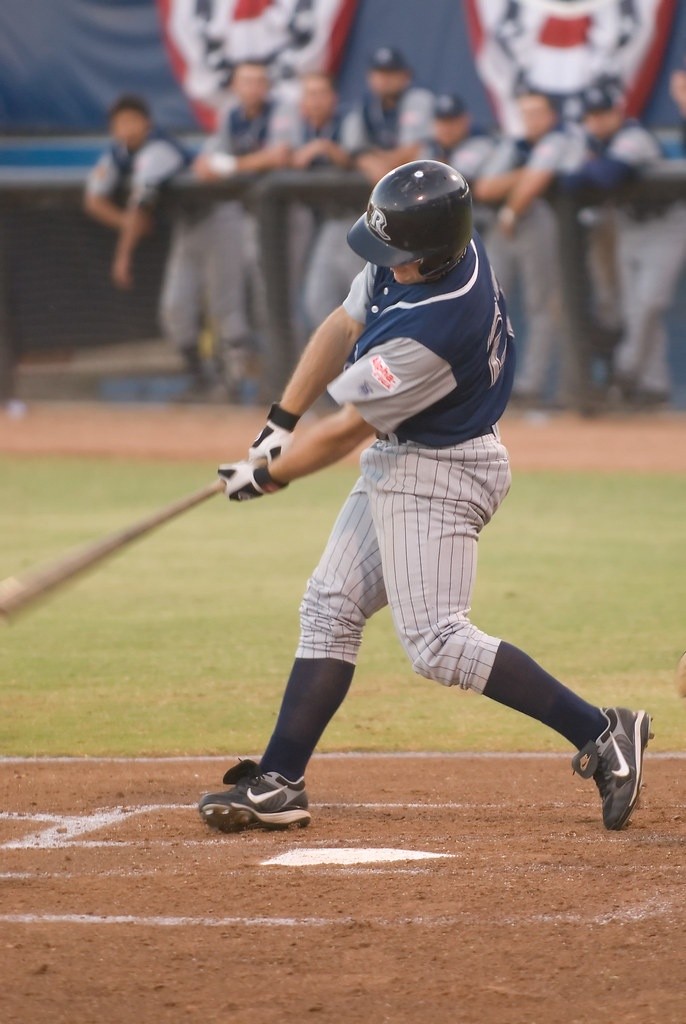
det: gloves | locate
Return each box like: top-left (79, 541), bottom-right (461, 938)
top-left (218, 462), bottom-right (289, 503)
top-left (246, 402), bottom-right (299, 467)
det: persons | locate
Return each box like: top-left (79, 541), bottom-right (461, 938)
top-left (190, 160), bottom-right (650, 832)
top-left (85, 47), bottom-right (686, 426)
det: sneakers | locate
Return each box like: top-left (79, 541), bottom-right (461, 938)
top-left (570, 706), bottom-right (655, 831)
top-left (198, 760), bottom-right (311, 835)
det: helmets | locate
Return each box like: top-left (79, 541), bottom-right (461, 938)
top-left (345, 160), bottom-right (471, 278)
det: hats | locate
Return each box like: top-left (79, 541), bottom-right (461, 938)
top-left (435, 92), bottom-right (464, 120)
top-left (369, 46), bottom-right (404, 72)
top-left (576, 89), bottom-right (610, 125)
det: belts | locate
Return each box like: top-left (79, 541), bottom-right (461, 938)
top-left (375, 426), bottom-right (493, 443)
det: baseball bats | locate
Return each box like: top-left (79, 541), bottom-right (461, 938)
top-left (0, 456), bottom-right (266, 618)
